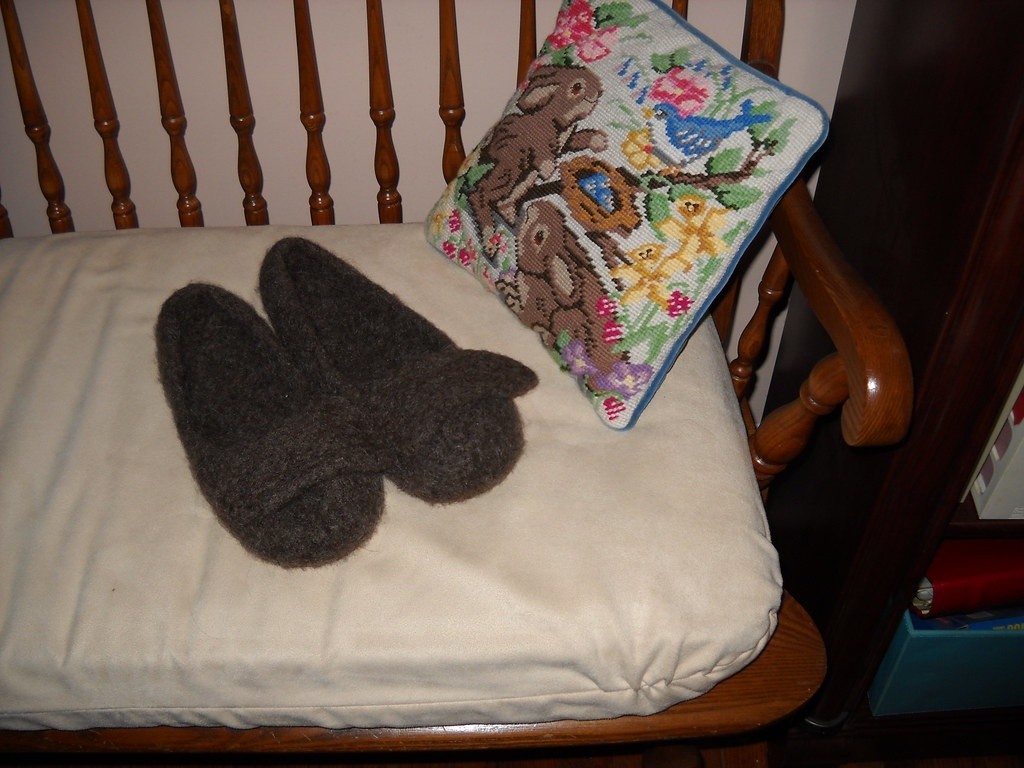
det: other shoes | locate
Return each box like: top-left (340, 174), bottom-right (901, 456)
top-left (156, 282), bottom-right (387, 570)
top-left (257, 236), bottom-right (537, 507)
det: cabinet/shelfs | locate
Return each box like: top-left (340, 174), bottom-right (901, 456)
top-left (763, 0), bottom-right (1024, 768)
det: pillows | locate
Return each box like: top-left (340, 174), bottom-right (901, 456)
top-left (427, 0), bottom-right (835, 433)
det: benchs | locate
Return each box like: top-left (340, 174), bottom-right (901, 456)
top-left (0, 0), bottom-right (919, 768)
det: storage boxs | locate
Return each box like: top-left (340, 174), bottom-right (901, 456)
top-left (870, 602), bottom-right (1024, 718)
top-left (972, 388), bottom-right (1024, 520)
